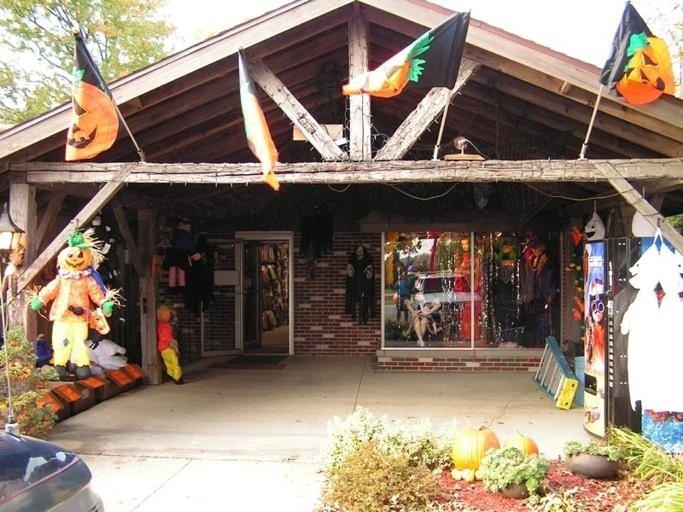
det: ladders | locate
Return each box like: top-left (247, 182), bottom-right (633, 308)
top-left (532, 336), bottom-right (578, 409)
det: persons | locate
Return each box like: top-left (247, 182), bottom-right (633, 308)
top-left (395, 271), bottom-right (409, 325)
top-left (156, 303), bottom-right (185, 385)
top-left (586, 294), bottom-right (605, 376)
top-left (404, 276), bottom-right (441, 346)
top-left (454, 236), bottom-right (482, 337)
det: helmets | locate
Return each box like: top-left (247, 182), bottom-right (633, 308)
top-left (408, 265), bottom-right (416, 270)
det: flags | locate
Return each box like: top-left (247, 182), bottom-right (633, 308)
top-left (599, 1), bottom-right (675, 109)
top-left (62, 31), bottom-right (125, 163)
top-left (341, 4), bottom-right (469, 100)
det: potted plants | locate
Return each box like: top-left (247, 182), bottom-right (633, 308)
top-left (563, 442), bottom-right (621, 478)
top-left (481, 447), bottom-right (549, 506)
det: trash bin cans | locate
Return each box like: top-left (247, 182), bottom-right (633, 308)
top-left (574, 356), bottom-right (584, 407)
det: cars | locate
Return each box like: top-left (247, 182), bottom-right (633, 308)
top-left (399, 269), bottom-right (482, 333)
top-left (0, 429), bottom-right (103, 511)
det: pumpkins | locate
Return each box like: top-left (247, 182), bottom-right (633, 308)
top-left (451, 426), bottom-right (499, 471)
top-left (505, 429), bottom-right (540, 458)
